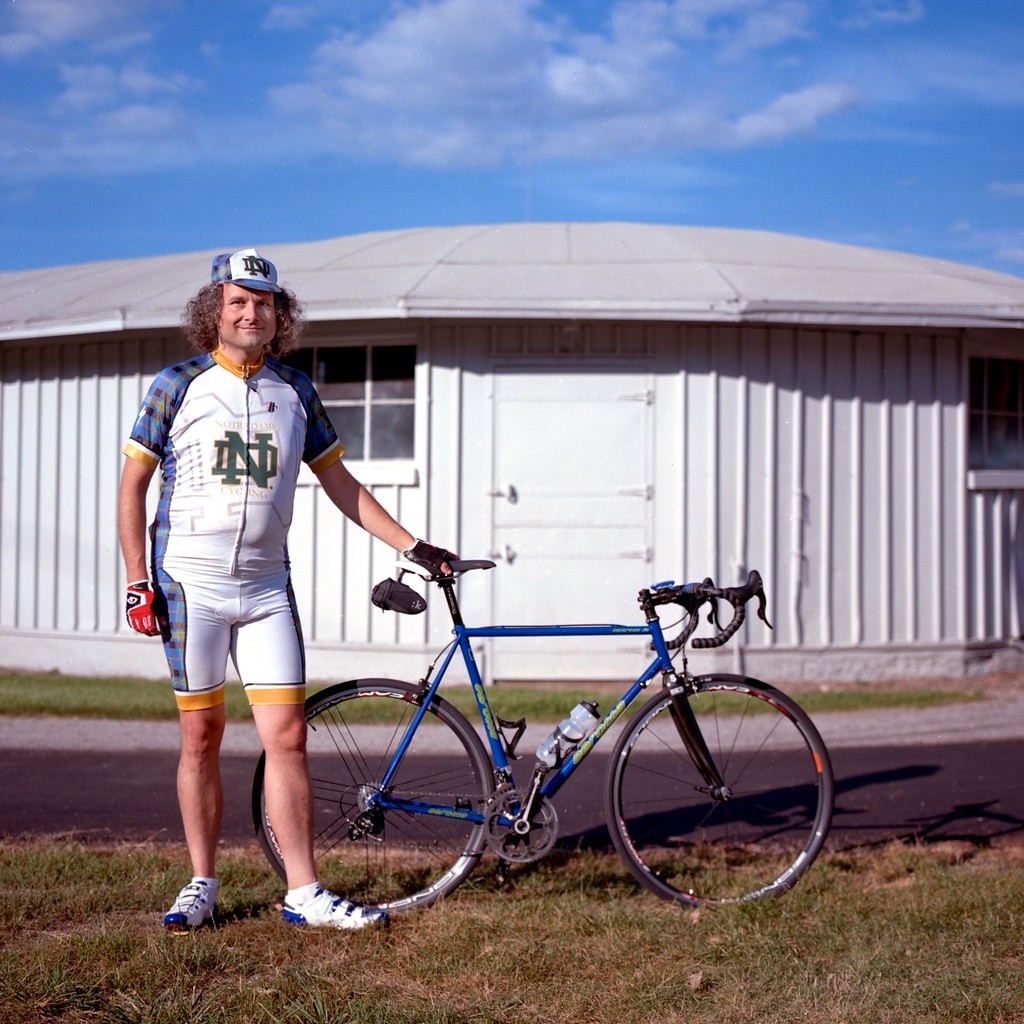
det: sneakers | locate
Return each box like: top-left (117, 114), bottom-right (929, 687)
top-left (283, 887), bottom-right (390, 932)
top-left (164, 882), bottom-right (219, 935)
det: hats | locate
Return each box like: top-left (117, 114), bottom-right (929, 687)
top-left (210, 248), bottom-right (283, 293)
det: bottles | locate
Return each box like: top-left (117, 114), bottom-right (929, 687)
top-left (535, 700), bottom-right (602, 767)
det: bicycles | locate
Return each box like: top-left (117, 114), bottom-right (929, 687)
top-left (252, 558), bottom-right (835, 911)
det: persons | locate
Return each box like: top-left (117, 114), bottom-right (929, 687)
top-left (114, 248), bottom-right (453, 939)
